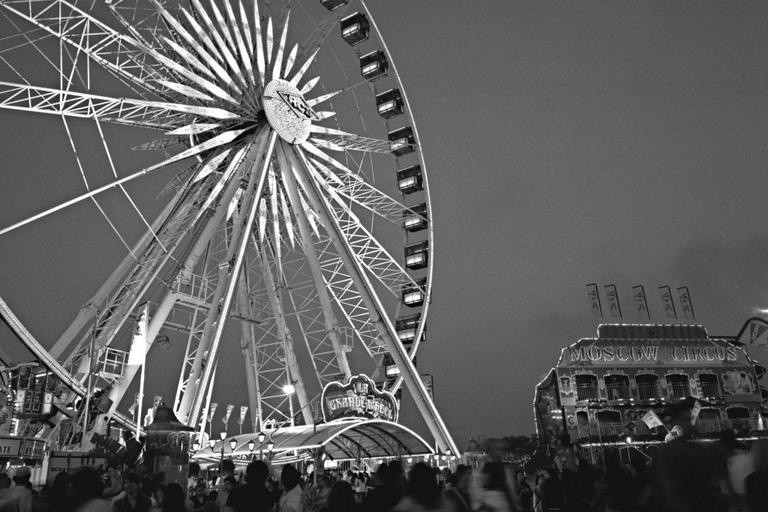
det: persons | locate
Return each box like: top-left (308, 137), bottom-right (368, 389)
top-left (1, 428), bottom-right (767, 511)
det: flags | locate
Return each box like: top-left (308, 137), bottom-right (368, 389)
top-left (151, 394), bottom-right (162, 418)
top-left (237, 406), bottom-right (249, 424)
top-left (207, 404), bottom-right (219, 422)
top-left (222, 403), bottom-right (235, 423)
top-left (128, 392), bottom-right (145, 414)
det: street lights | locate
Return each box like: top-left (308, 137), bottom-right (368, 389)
top-left (188, 440), bottom-right (200, 460)
top-left (281, 385), bottom-right (299, 458)
top-left (209, 402), bottom-right (219, 438)
top-left (209, 432), bottom-right (237, 474)
top-left (253, 407), bottom-right (262, 432)
top-left (246, 433), bottom-right (274, 463)
top-left (238, 406), bottom-right (248, 433)
top-left (223, 404), bottom-right (235, 432)
